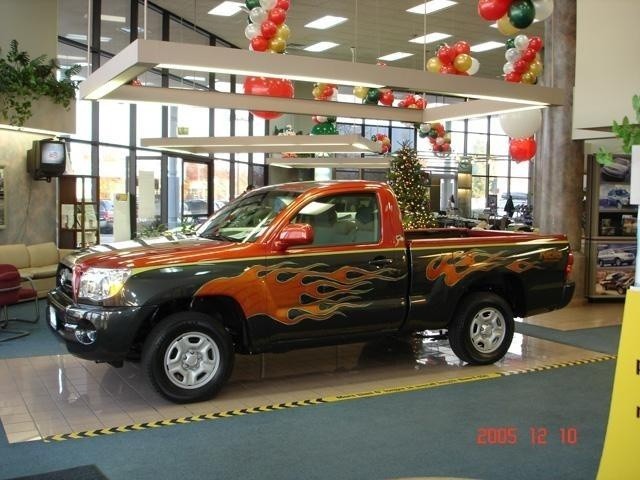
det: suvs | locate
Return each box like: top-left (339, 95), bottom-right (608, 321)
top-left (607, 188), bottom-right (635, 208)
top-left (598, 249), bottom-right (635, 267)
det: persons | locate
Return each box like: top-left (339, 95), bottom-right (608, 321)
top-left (504, 196), bottom-right (515, 218)
top-left (236, 185), bottom-right (255, 198)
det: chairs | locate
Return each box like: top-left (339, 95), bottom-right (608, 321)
top-left (0, 263), bottom-right (42, 329)
top-left (0, 270), bottom-right (31, 342)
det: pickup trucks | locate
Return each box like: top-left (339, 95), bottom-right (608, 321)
top-left (46, 180), bottom-right (575, 405)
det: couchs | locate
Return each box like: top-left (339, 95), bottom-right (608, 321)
top-left (0, 241), bottom-right (83, 304)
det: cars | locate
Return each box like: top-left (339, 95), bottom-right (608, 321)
top-left (600, 157), bottom-right (631, 182)
top-left (98, 197), bottom-right (230, 234)
top-left (599, 272), bottom-right (634, 295)
top-left (497, 191), bottom-right (528, 212)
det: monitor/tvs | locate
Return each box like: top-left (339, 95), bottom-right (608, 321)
top-left (27, 140), bottom-right (66, 177)
